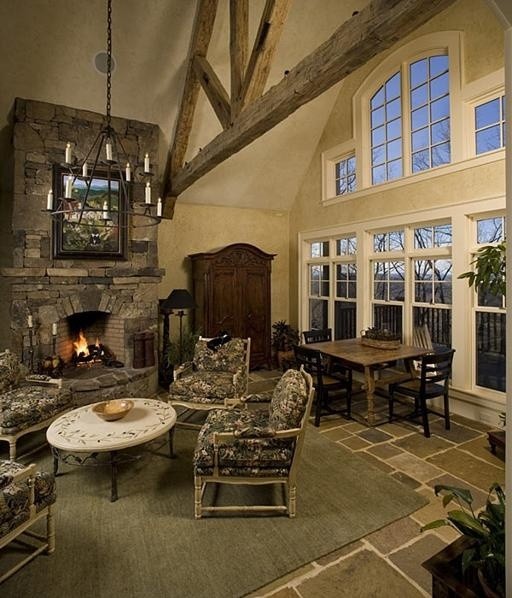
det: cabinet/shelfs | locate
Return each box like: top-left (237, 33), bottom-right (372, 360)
top-left (185, 241), bottom-right (280, 372)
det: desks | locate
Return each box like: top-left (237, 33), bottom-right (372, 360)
top-left (300, 338), bottom-right (435, 429)
top-left (44, 397), bottom-right (178, 502)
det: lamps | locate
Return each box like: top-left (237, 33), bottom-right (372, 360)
top-left (162, 288), bottom-right (199, 367)
top-left (41, 0), bottom-right (166, 230)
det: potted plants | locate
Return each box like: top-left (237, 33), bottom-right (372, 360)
top-left (413, 475), bottom-right (507, 598)
top-left (270, 315), bottom-right (301, 371)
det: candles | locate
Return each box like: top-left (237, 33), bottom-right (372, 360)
top-left (26, 312), bottom-right (33, 326)
top-left (51, 319), bottom-right (57, 336)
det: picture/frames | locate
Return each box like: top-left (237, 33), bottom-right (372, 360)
top-left (48, 163), bottom-right (131, 263)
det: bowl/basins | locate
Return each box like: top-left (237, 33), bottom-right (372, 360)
top-left (92, 397), bottom-right (136, 422)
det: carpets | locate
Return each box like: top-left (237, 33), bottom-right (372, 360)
top-left (1, 426), bottom-right (429, 598)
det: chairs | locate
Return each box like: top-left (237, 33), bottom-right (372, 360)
top-left (384, 348), bottom-right (458, 439)
top-left (166, 332), bottom-right (252, 432)
top-left (293, 342), bottom-right (353, 430)
top-left (301, 328), bottom-right (348, 378)
top-left (0, 454), bottom-right (61, 585)
top-left (0, 348), bottom-right (73, 467)
top-left (183, 363), bottom-right (317, 519)
